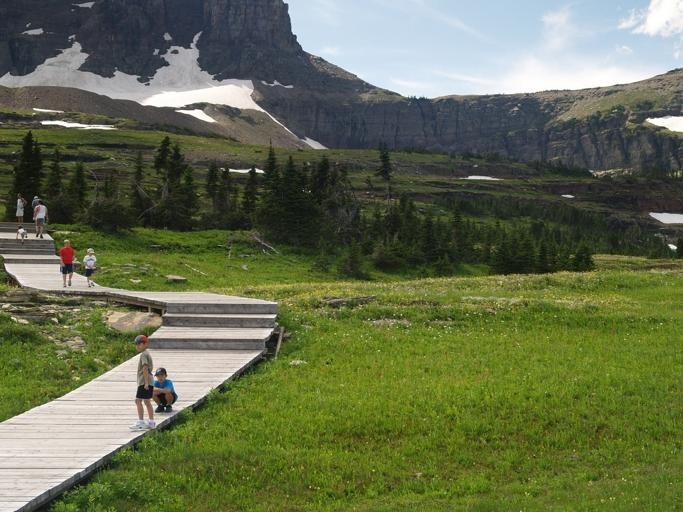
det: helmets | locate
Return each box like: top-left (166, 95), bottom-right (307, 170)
top-left (134, 335), bottom-right (148, 344)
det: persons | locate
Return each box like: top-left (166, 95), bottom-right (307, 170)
top-left (32, 199), bottom-right (48, 239)
top-left (83, 247), bottom-right (97, 287)
top-left (151, 366), bottom-right (177, 414)
top-left (128, 334), bottom-right (157, 432)
top-left (14, 193), bottom-right (28, 223)
top-left (15, 225), bottom-right (28, 246)
top-left (58, 239), bottom-right (76, 287)
top-left (30, 196), bottom-right (41, 210)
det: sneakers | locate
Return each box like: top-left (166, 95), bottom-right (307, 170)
top-left (36, 232), bottom-right (43, 238)
top-left (128, 423), bottom-right (144, 431)
top-left (88, 282), bottom-right (94, 287)
top-left (154, 405), bottom-right (164, 412)
top-left (143, 422), bottom-right (156, 429)
top-left (165, 404), bottom-right (172, 411)
top-left (62, 282), bottom-right (72, 287)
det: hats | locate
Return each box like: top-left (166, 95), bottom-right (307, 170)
top-left (87, 248), bottom-right (95, 254)
top-left (154, 368), bottom-right (166, 376)
